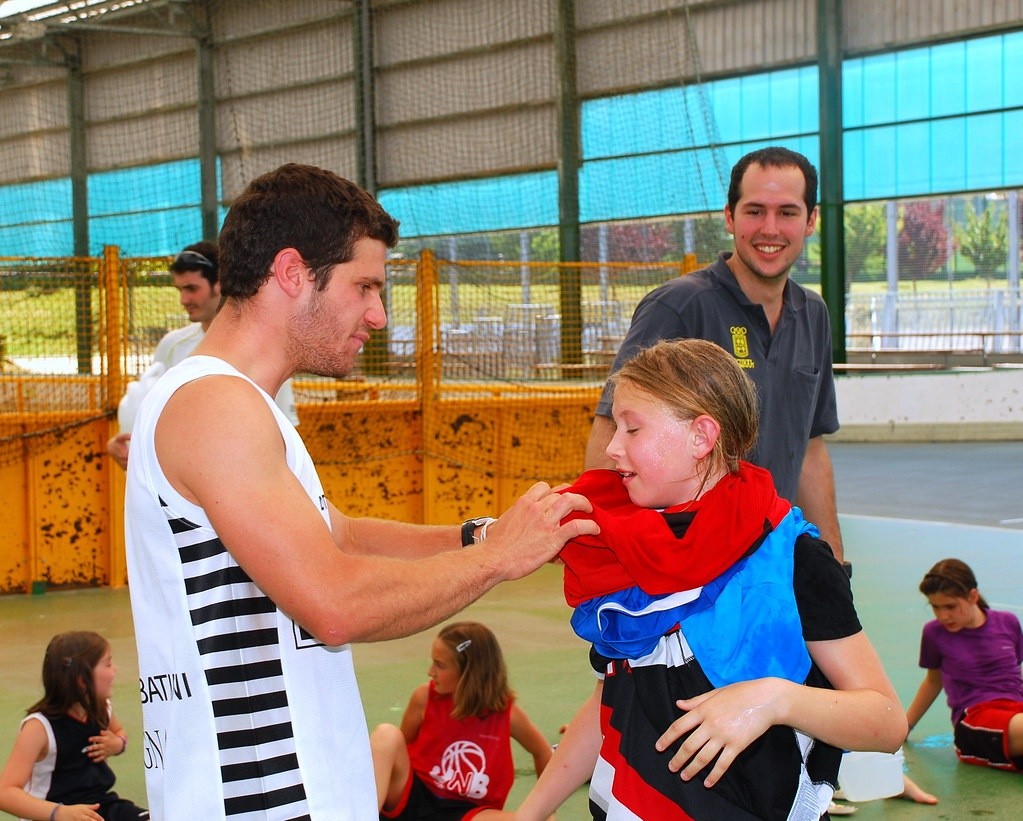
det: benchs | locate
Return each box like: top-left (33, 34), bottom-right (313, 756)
top-left (536, 362), bottom-right (612, 375)
top-left (595, 334), bottom-right (627, 342)
top-left (580, 349), bottom-right (621, 359)
top-left (831, 361), bottom-right (948, 371)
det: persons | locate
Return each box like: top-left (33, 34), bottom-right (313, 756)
top-left (371, 622), bottom-right (560, 821)
top-left (102, 242), bottom-right (305, 472)
top-left (897, 556), bottom-right (1021, 772)
top-left (124, 158), bottom-right (599, 818)
top-left (517, 339), bottom-right (912, 817)
top-left (2, 631), bottom-right (148, 818)
top-left (585, 149), bottom-right (851, 816)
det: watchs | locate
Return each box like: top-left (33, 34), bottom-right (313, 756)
top-left (455, 513), bottom-right (494, 547)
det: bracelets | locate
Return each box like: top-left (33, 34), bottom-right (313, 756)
top-left (479, 518), bottom-right (497, 540)
